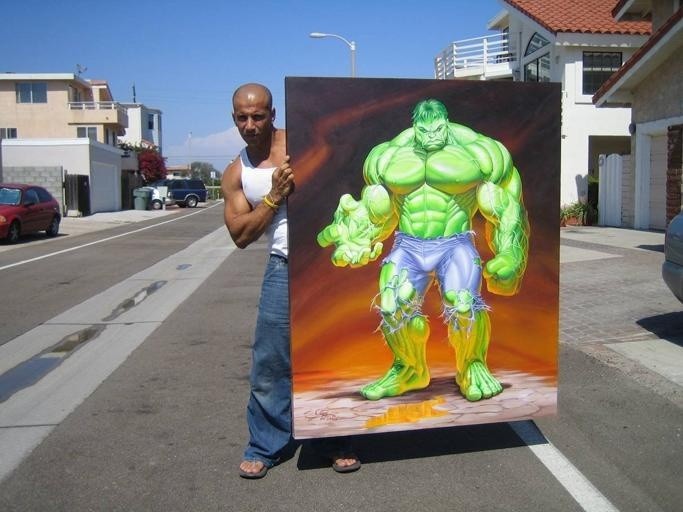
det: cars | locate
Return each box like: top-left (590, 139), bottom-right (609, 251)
top-left (662, 208), bottom-right (683, 304)
top-left (0, 182), bottom-right (61, 242)
top-left (143, 179), bottom-right (208, 209)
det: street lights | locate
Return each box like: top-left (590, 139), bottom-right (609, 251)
top-left (309, 33), bottom-right (356, 77)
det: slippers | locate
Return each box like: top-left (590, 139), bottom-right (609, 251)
top-left (238, 459), bottom-right (268, 479)
top-left (332, 454), bottom-right (360, 472)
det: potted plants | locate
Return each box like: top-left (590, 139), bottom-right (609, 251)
top-left (560, 200), bottom-right (597, 228)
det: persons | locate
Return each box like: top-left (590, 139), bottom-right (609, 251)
top-left (317, 97), bottom-right (531, 402)
top-left (219, 83), bottom-right (361, 478)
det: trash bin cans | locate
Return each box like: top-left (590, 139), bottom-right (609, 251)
top-left (133, 189), bottom-right (152, 210)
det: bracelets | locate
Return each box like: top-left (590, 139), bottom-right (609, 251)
top-left (264, 196), bottom-right (279, 209)
